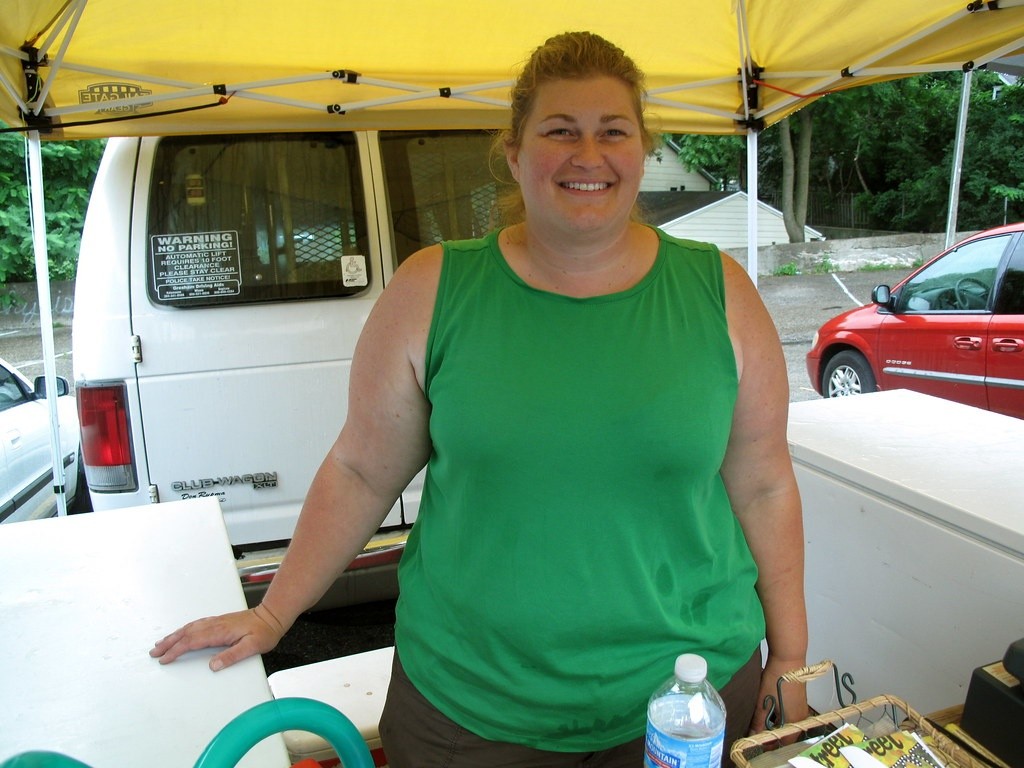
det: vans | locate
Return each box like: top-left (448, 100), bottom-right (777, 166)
top-left (67, 128), bottom-right (531, 616)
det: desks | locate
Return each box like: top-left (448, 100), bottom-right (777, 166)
top-left (0, 493), bottom-right (291, 767)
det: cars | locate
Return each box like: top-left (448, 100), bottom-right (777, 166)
top-left (0, 361), bottom-right (90, 525)
top-left (801, 224), bottom-right (1022, 422)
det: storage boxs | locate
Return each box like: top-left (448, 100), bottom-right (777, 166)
top-left (729, 661), bottom-right (989, 768)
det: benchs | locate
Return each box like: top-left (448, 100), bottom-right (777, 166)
top-left (268, 644), bottom-right (396, 768)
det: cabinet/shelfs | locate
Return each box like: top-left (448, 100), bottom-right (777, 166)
top-left (761, 388), bottom-right (1024, 732)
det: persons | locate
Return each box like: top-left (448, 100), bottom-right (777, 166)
top-left (151, 33), bottom-right (808, 767)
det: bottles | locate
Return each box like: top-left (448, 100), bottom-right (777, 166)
top-left (643, 652), bottom-right (727, 768)
